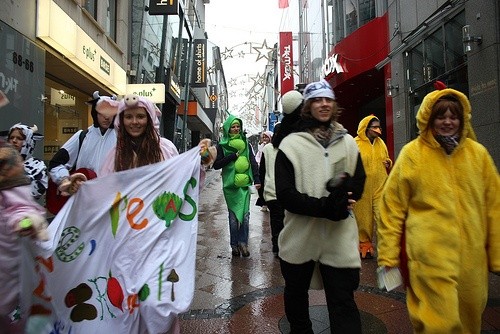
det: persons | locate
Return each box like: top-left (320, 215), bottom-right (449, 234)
top-left (50, 91), bottom-right (118, 188)
top-left (378, 81), bottom-right (500, 334)
top-left (274, 81), bottom-right (367, 334)
top-left (0, 124), bottom-right (46, 334)
top-left (71, 95), bottom-right (217, 334)
top-left (213, 115), bottom-right (261, 258)
top-left (255, 91), bottom-right (304, 260)
top-left (354, 114), bottom-right (392, 259)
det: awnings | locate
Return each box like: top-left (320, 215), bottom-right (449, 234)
top-left (323, 16), bottom-right (387, 90)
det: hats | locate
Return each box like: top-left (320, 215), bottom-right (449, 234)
top-left (303, 82), bottom-right (335, 103)
top-left (282, 90), bottom-right (303, 114)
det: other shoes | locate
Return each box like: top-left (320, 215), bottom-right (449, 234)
top-left (231, 245), bottom-right (240, 259)
top-left (240, 243), bottom-right (251, 258)
top-left (261, 206), bottom-right (267, 212)
